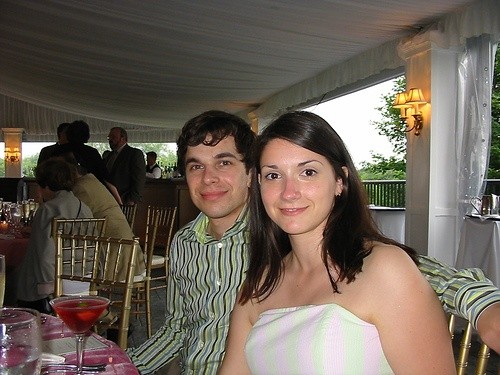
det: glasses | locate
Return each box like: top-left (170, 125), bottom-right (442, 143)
top-left (106, 135), bottom-right (121, 140)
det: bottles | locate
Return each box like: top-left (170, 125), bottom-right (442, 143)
top-left (157, 161), bottom-right (179, 179)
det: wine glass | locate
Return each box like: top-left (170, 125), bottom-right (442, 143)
top-left (49, 295), bottom-right (111, 375)
top-left (0, 198), bottom-right (35, 235)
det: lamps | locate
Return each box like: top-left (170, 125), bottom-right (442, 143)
top-left (3, 146), bottom-right (22, 165)
top-left (390, 88), bottom-right (429, 135)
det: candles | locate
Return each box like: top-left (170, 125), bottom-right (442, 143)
top-left (0, 221), bottom-right (9, 234)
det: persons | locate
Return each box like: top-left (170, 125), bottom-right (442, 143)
top-left (37, 121), bottom-right (102, 180)
top-left (51, 152), bottom-right (143, 334)
top-left (102, 127), bottom-right (145, 204)
top-left (7, 159), bottom-right (98, 301)
top-left (217, 111), bottom-right (457, 375)
top-left (123, 110), bottom-right (500, 375)
top-left (145, 152), bottom-right (162, 179)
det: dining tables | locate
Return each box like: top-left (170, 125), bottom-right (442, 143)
top-left (0, 308), bottom-right (138, 375)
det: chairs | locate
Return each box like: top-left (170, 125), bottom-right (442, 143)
top-left (51, 197), bottom-right (179, 349)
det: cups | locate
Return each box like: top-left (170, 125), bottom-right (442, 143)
top-left (0, 221), bottom-right (9, 234)
top-left (0, 254), bottom-right (6, 308)
top-left (0, 308), bottom-right (41, 375)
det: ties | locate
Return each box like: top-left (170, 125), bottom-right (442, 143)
top-left (108, 152), bottom-right (116, 168)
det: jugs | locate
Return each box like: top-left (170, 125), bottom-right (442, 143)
top-left (470, 194), bottom-right (500, 215)
